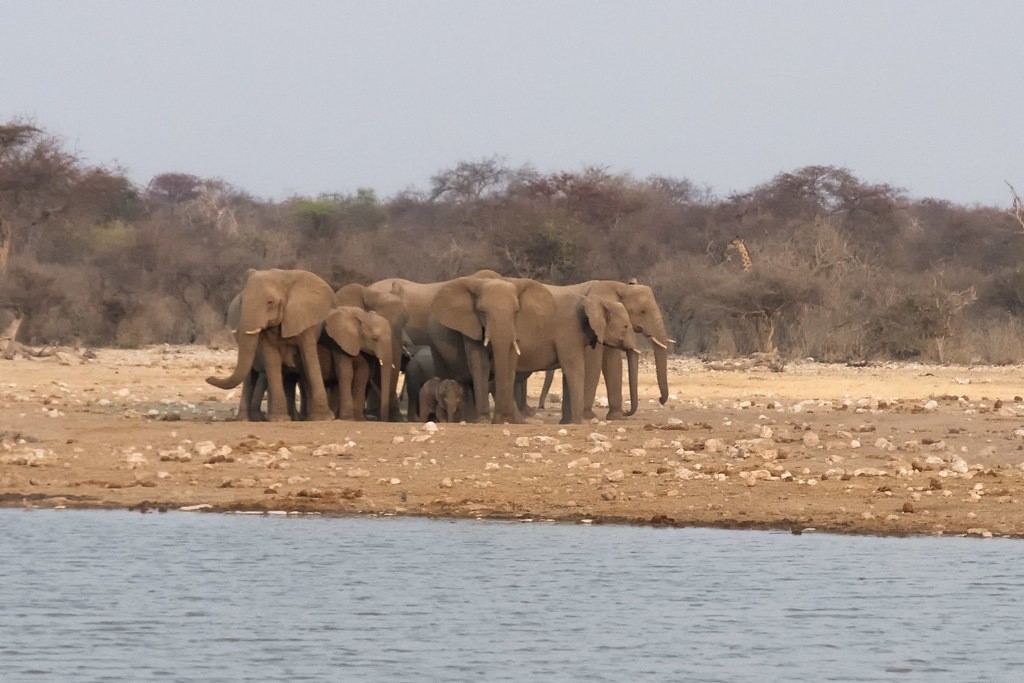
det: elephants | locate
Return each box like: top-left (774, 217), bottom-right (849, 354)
top-left (205, 267), bottom-right (676, 424)
